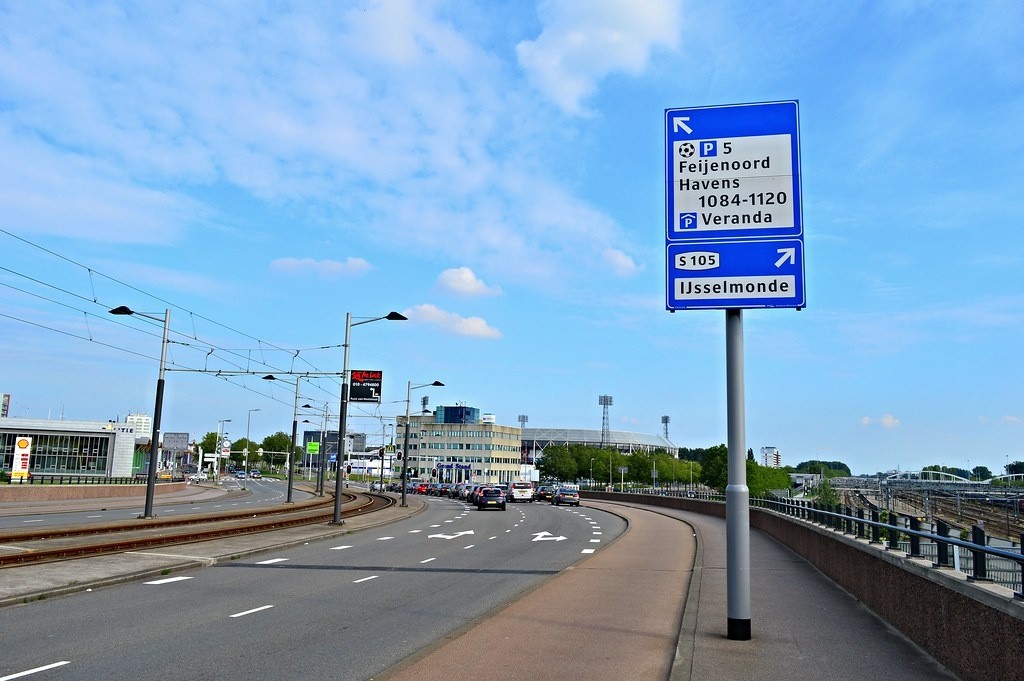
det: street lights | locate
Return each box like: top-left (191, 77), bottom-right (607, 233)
top-left (649, 458), bottom-right (660, 489)
top-left (685, 460), bottom-right (699, 491)
top-left (1006, 454), bottom-right (1010, 486)
top-left (213, 418), bottom-right (232, 479)
top-left (217, 432), bottom-right (229, 459)
top-left (261, 374), bottom-right (300, 507)
top-left (301, 404), bottom-right (329, 497)
top-left (388, 423), bottom-right (394, 473)
top-left (420, 395), bottom-right (429, 416)
top-left (107, 306), bottom-right (171, 519)
top-left (590, 458), bottom-right (595, 492)
top-left (518, 415), bottom-right (529, 428)
top-left (662, 415), bottom-right (670, 439)
top-left (967, 458), bottom-right (971, 487)
top-left (598, 393), bottom-right (613, 450)
top-left (244, 408), bottom-right (261, 490)
top-left (302, 420), bottom-right (323, 493)
top-left (329, 311), bottom-right (408, 525)
top-left (398, 379), bottom-right (446, 507)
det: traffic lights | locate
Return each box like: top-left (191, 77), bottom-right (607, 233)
top-left (432, 468), bottom-right (436, 477)
top-left (347, 466), bottom-right (351, 473)
top-left (379, 449), bottom-right (383, 457)
top-left (397, 452), bottom-right (401, 460)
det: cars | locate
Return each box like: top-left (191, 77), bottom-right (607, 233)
top-left (184, 469), bottom-right (191, 474)
top-left (253, 472), bottom-right (263, 479)
top-left (228, 463), bottom-right (245, 479)
top-left (387, 480), bottom-right (580, 511)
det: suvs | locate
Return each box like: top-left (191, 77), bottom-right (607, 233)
top-left (189, 472), bottom-right (207, 481)
top-left (370, 480), bottom-right (385, 492)
top-left (250, 469), bottom-right (260, 478)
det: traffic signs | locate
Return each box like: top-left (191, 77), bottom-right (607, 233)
top-left (665, 239), bottom-right (807, 310)
top-left (663, 97), bottom-right (804, 242)
top-left (350, 369), bottom-right (383, 403)
top-left (307, 442), bottom-right (319, 454)
